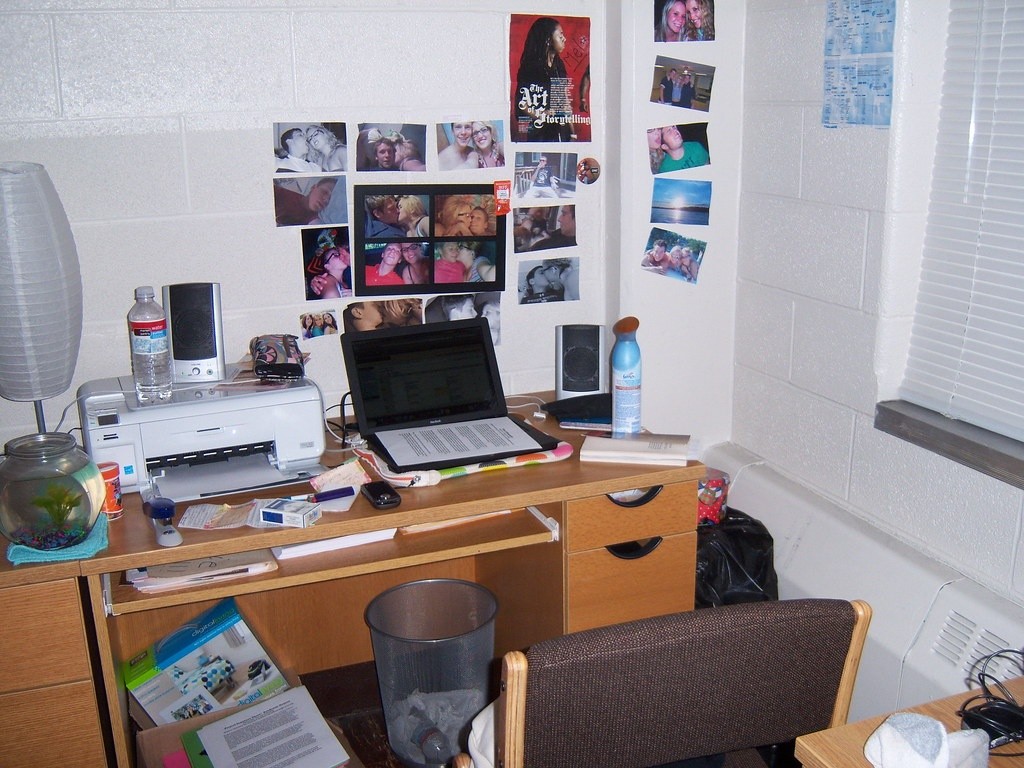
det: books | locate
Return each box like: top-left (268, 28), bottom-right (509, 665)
top-left (543, 392), bottom-right (692, 466)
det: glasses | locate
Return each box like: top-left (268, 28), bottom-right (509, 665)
top-left (307, 129), bottom-right (325, 143)
top-left (401, 244), bottom-right (422, 253)
top-left (539, 160), bottom-right (546, 162)
top-left (647, 127), bottom-right (663, 133)
top-left (471, 125), bottom-right (489, 138)
top-left (457, 213), bottom-right (472, 218)
top-left (671, 254), bottom-right (681, 260)
top-left (682, 254), bottom-right (690, 260)
top-left (322, 252), bottom-right (340, 265)
top-left (459, 244), bottom-right (472, 251)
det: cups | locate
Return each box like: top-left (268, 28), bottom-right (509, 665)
top-left (96, 462), bottom-right (125, 522)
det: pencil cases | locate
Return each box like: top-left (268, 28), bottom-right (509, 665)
top-left (249, 333), bottom-right (305, 382)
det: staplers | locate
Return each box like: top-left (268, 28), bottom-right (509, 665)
top-left (142, 497), bottom-right (184, 548)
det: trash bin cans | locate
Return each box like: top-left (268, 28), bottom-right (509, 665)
top-left (363, 577), bottom-right (499, 768)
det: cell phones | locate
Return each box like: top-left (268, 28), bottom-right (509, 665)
top-left (361, 480), bottom-right (401, 509)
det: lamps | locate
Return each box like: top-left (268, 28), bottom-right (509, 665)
top-left (0, 161), bottom-right (85, 433)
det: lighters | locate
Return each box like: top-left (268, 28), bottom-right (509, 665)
top-left (307, 486), bottom-right (354, 502)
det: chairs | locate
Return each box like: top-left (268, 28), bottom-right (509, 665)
top-left (453, 600), bottom-right (871, 768)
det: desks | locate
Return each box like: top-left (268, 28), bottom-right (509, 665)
top-left (0, 390), bottom-right (705, 768)
top-left (795, 674), bottom-right (1024, 768)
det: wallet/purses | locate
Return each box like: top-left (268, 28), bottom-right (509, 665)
top-left (251, 331), bottom-right (306, 383)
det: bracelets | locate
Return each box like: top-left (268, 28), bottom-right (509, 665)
top-left (581, 99), bottom-right (586, 101)
top-left (570, 135), bottom-right (577, 139)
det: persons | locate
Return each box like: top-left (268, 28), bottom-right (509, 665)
top-left (647, 125), bottom-right (709, 173)
top-left (438, 121), bottom-right (505, 171)
top-left (641, 240), bottom-right (704, 280)
top-left (300, 312), bottom-right (337, 339)
top-left (514, 205), bottom-right (576, 252)
top-left (656, 0), bottom-right (714, 41)
top-left (366, 243), bottom-right (430, 286)
top-left (274, 125), bottom-right (346, 173)
top-left (344, 293), bottom-right (500, 346)
top-left (658, 69), bottom-right (695, 109)
top-left (518, 258), bottom-right (579, 304)
top-left (579, 65), bottom-right (589, 112)
top-left (525, 156), bottom-right (559, 199)
top-left (515, 18), bottom-right (577, 142)
top-left (275, 177), bottom-right (337, 225)
top-left (435, 196), bottom-right (496, 283)
top-left (365, 194), bottom-right (429, 238)
top-left (310, 229), bottom-right (352, 299)
top-left (374, 138), bottom-right (425, 171)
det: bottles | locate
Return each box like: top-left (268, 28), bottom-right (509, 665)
top-left (408, 705), bottom-right (454, 764)
top-left (611, 314), bottom-right (643, 435)
top-left (127, 286), bottom-right (174, 404)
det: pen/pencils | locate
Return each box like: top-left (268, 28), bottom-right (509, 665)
top-left (280, 494), bottom-right (316, 501)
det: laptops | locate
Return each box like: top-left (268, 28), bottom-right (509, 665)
top-left (341, 317), bottom-right (557, 475)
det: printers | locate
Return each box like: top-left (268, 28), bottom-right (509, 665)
top-left (76, 361), bottom-right (333, 504)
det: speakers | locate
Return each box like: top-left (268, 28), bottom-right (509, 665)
top-left (161, 282), bottom-right (227, 384)
top-left (555, 324), bottom-right (606, 401)
